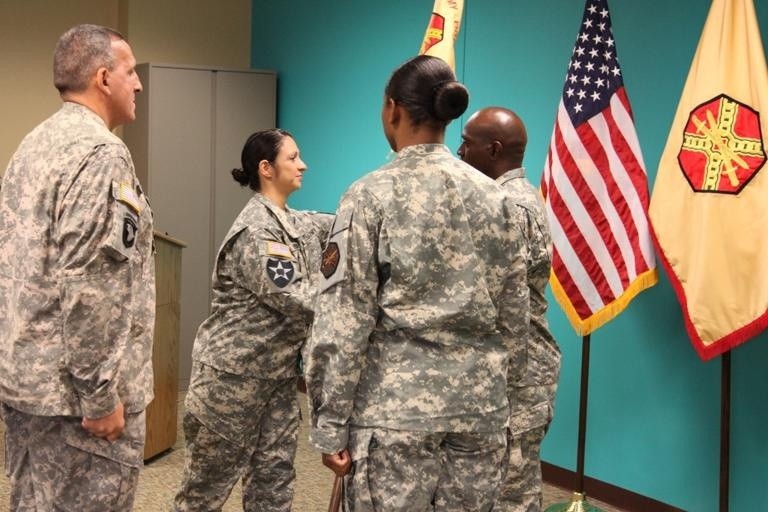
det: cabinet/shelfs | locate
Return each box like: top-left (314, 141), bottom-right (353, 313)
top-left (122, 59), bottom-right (280, 391)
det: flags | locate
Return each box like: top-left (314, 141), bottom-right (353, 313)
top-left (421, 1), bottom-right (463, 83)
top-left (540, 2), bottom-right (657, 340)
top-left (646, 0), bottom-right (768, 366)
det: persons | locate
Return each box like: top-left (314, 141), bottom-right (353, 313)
top-left (456, 106), bottom-right (561, 511)
top-left (2, 23), bottom-right (160, 509)
top-left (308, 56), bottom-right (531, 511)
top-left (171, 125), bottom-right (338, 511)
